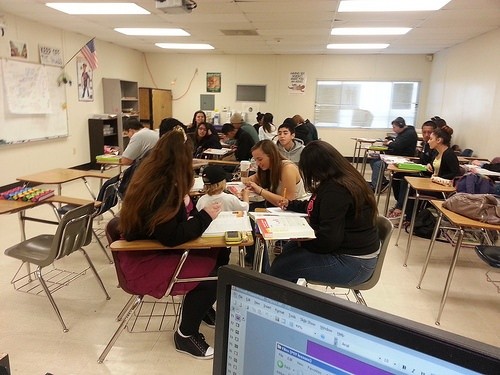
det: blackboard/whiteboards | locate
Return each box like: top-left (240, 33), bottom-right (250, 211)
top-left (0, 57), bottom-right (70, 145)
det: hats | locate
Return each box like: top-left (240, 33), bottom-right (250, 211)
top-left (202, 165), bottom-right (233, 185)
top-left (230, 113), bottom-right (245, 124)
top-left (159, 118), bottom-right (187, 138)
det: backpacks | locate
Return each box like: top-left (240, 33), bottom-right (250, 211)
top-left (456, 173), bottom-right (495, 194)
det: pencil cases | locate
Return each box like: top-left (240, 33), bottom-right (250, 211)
top-left (225, 231), bottom-right (243, 244)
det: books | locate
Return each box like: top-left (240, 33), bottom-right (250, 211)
top-left (202, 210), bottom-right (253, 237)
top-left (248, 211), bottom-right (317, 240)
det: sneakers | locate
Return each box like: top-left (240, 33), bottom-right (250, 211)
top-left (202, 307), bottom-right (215, 327)
top-left (174, 331), bottom-right (214, 359)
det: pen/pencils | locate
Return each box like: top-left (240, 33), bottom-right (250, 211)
top-left (282, 188), bottom-right (286, 210)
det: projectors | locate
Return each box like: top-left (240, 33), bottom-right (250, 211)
top-left (156, 0), bottom-right (193, 14)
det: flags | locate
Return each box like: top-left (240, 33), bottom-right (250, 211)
top-left (80, 39), bottom-right (99, 71)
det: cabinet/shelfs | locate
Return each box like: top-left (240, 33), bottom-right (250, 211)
top-left (88, 118), bottom-right (119, 170)
top-left (102, 78), bottom-right (139, 155)
top-left (138, 87), bottom-right (172, 131)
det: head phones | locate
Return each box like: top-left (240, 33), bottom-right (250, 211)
top-left (395, 120), bottom-right (403, 128)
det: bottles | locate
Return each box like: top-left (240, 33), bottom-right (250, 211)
top-left (229, 107), bottom-right (232, 122)
top-left (214, 112), bottom-right (219, 125)
top-left (222, 106), bottom-right (227, 112)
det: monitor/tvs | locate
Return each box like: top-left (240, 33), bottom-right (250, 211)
top-left (211, 265), bottom-right (500, 375)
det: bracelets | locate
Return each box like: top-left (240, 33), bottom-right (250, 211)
top-left (259, 189), bottom-right (264, 195)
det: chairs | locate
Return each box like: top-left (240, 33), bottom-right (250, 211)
top-left (0, 133), bottom-right (500, 364)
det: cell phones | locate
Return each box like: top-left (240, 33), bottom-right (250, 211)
top-left (225, 231), bottom-right (241, 242)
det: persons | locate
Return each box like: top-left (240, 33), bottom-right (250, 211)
top-left (369, 116), bottom-right (459, 228)
top-left (121, 117), bottom-right (159, 166)
top-left (270, 140), bottom-right (382, 287)
top-left (160, 110), bottom-right (319, 274)
top-left (472, 159), bottom-right (500, 173)
top-left (119, 125), bottom-right (231, 360)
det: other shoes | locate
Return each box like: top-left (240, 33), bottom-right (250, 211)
top-left (389, 203), bottom-right (402, 218)
top-left (372, 182), bottom-right (390, 193)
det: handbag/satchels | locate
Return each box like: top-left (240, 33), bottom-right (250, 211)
top-left (442, 191), bottom-right (500, 224)
top-left (405, 209), bottom-right (441, 239)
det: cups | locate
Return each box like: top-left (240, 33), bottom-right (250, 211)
top-left (219, 112), bottom-right (230, 125)
top-left (103, 124), bottom-right (113, 134)
top-left (241, 160), bottom-right (251, 182)
